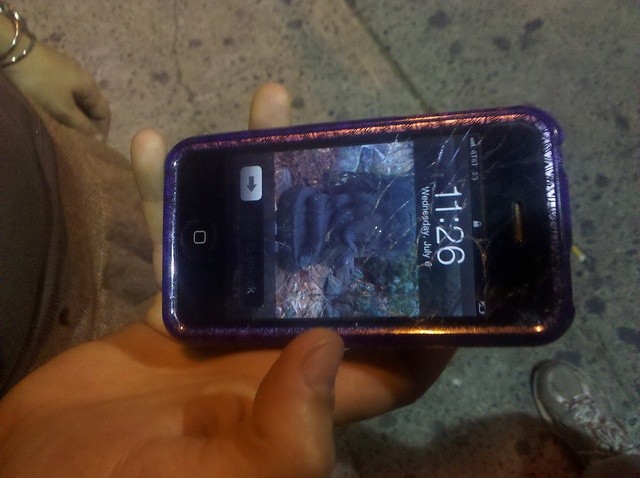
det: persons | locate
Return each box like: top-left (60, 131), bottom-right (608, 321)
top-left (2, 8), bottom-right (458, 478)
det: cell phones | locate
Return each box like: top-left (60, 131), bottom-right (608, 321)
top-left (162, 104), bottom-right (575, 350)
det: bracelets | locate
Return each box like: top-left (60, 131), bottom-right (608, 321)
top-left (0, 3), bottom-right (35, 67)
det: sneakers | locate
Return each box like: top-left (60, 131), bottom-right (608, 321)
top-left (530, 356), bottom-right (636, 466)
